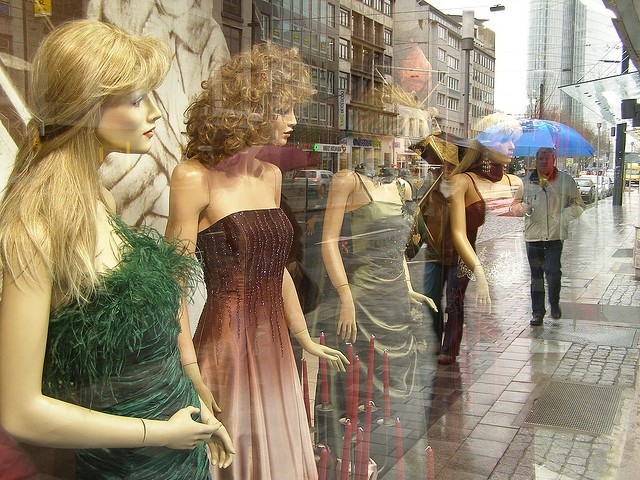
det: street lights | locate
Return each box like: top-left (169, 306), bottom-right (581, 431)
top-left (595, 116), bottom-right (602, 205)
top-left (462, 10), bottom-right (476, 138)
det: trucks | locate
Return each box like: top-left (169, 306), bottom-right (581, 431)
top-left (623, 161), bottom-right (640, 186)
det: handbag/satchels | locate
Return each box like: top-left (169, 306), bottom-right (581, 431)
top-left (287, 254), bottom-right (323, 314)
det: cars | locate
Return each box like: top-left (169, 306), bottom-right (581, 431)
top-left (569, 178), bottom-right (595, 203)
top-left (603, 177), bottom-right (615, 196)
top-left (292, 169), bottom-right (339, 200)
top-left (581, 175), bottom-right (607, 199)
top-left (355, 159), bottom-right (406, 180)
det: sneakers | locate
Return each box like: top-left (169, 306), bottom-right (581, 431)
top-left (529, 315), bottom-right (543, 325)
top-left (430, 343), bottom-right (441, 355)
top-left (551, 303), bottom-right (562, 319)
top-left (437, 354), bottom-right (456, 365)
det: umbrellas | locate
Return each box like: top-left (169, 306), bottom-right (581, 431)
top-left (406, 129), bottom-right (503, 208)
top-left (472, 116), bottom-right (597, 218)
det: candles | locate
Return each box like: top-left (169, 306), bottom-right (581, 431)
top-left (301, 357), bottom-right (311, 428)
top-left (320, 331), bottom-right (329, 405)
top-left (351, 355), bottom-right (360, 432)
top-left (340, 419), bottom-right (352, 480)
top-left (318, 445), bottom-right (327, 480)
top-left (395, 418), bottom-right (405, 480)
top-left (383, 349), bottom-right (390, 417)
top-left (355, 427), bottom-right (363, 480)
top-left (346, 343), bottom-right (354, 419)
top-left (360, 401), bottom-right (375, 480)
top-left (366, 334), bottom-right (375, 410)
top-left (425, 446), bottom-right (435, 480)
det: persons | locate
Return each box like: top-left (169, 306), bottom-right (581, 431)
top-left (508, 146), bottom-right (586, 327)
top-left (165, 39), bottom-right (350, 480)
top-left (0, 25), bottom-right (237, 480)
top-left (428, 112), bottom-right (536, 479)
top-left (320, 80), bottom-right (439, 480)
top-left (86, 0), bottom-right (232, 230)
top-left (404, 162), bottom-right (472, 365)
